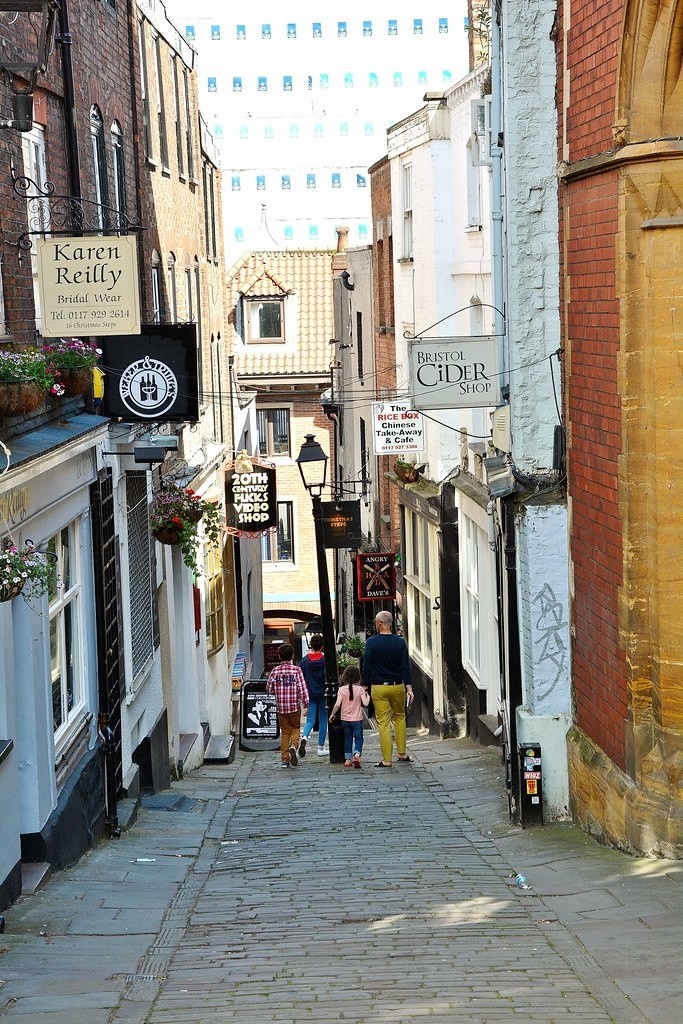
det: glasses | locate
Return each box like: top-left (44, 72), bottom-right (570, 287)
top-left (373, 619), bottom-right (383, 623)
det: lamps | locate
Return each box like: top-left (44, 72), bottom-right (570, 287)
top-left (226, 449), bottom-right (254, 473)
top-left (102, 447), bottom-right (167, 464)
top-left (0, 0), bottom-right (61, 133)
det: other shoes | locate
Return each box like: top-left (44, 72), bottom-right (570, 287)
top-left (289, 747), bottom-right (298, 766)
top-left (281, 761), bottom-right (290, 768)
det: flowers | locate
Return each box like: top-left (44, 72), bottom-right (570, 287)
top-left (149, 487), bottom-right (231, 577)
top-left (0, 537), bottom-right (66, 621)
top-left (0, 345), bottom-right (66, 395)
top-left (41, 337), bottom-right (103, 370)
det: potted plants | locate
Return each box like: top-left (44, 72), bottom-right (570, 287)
top-left (341, 634), bottom-right (366, 658)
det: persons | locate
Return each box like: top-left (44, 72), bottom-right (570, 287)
top-left (362, 611), bottom-right (415, 768)
top-left (265, 644), bottom-right (309, 769)
top-left (299, 635), bottom-right (330, 758)
top-left (328, 664), bottom-right (370, 769)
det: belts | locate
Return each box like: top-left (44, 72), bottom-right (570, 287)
top-left (372, 681), bottom-right (402, 686)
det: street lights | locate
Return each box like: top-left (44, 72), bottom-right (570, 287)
top-left (294, 434), bottom-right (347, 766)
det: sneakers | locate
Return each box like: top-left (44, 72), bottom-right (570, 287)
top-left (298, 736), bottom-right (308, 758)
top-left (317, 748), bottom-right (330, 755)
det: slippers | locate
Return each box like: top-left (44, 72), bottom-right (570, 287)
top-left (353, 758), bottom-right (363, 768)
top-left (374, 761), bottom-right (391, 766)
top-left (396, 755), bottom-right (414, 762)
top-left (345, 761), bottom-right (354, 767)
